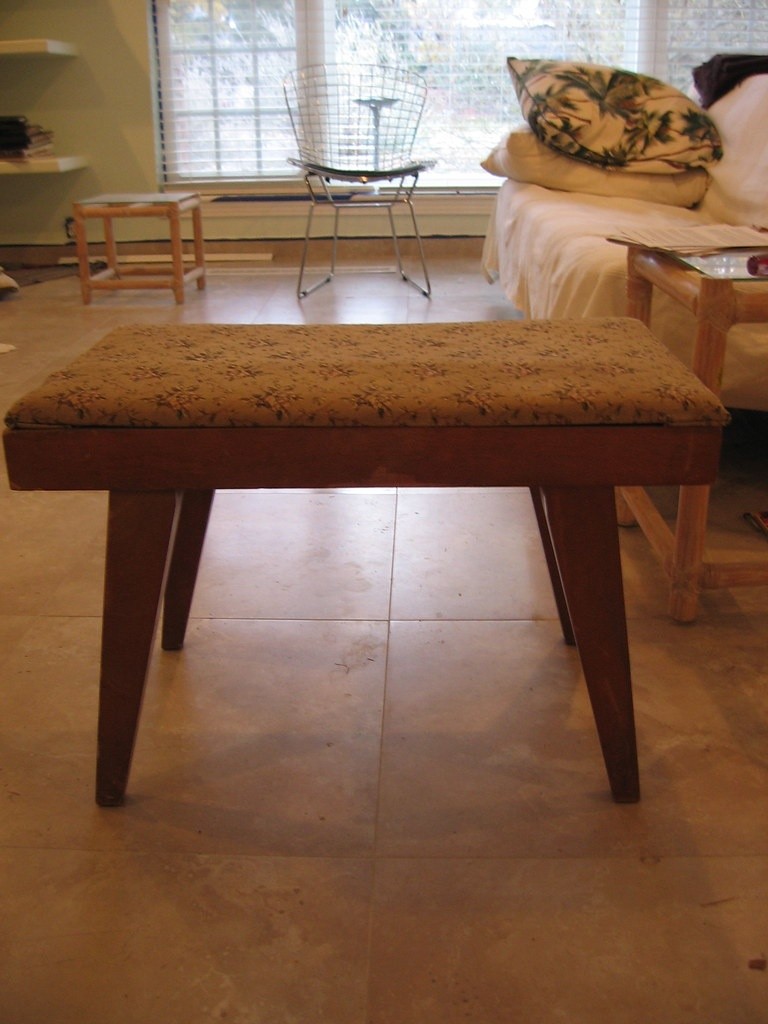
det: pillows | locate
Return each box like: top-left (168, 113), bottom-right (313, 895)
top-left (507, 56), bottom-right (723, 175)
top-left (479, 124), bottom-right (710, 208)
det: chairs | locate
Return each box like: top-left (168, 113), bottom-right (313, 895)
top-left (283, 62), bottom-right (435, 300)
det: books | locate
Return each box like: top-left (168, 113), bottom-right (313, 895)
top-left (0, 114), bottom-right (57, 160)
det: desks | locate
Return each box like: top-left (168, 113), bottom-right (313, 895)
top-left (614, 226), bottom-right (768, 621)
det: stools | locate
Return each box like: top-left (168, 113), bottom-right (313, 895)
top-left (71, 192), bottom-right (205, 305)
top-left (3, 316), bottom-right (727, 803)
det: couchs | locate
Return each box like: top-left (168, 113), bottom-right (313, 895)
top-left (479, 71), bottom-right (768, 411)
